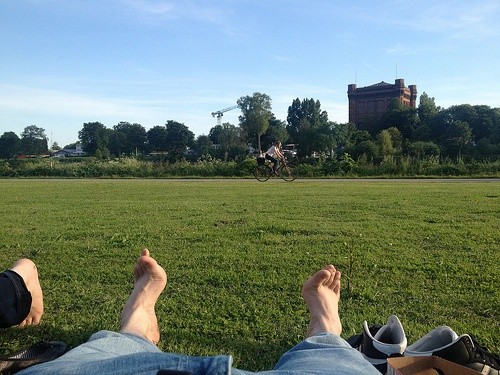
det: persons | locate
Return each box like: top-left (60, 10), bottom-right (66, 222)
top-left (13, 248), bottom-right (382, 375)
top-left (0, 259), bottom-right (42, 330)
top-left (266, 141), bottom-right (284, 176)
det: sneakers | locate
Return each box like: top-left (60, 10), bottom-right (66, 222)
top-left (345, 315), bottom-right (500, 375)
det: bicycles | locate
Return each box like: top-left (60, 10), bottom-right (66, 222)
top-left (253, 155), bottom-right (299, 183)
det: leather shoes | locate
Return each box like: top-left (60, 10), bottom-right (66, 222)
top-left (269, 170), bottom-right (280, 176)
top-left (0, 341), bottom-right (66, 375)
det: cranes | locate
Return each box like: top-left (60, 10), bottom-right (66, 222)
top-left (211, 106), bottom-right (239, 125)
